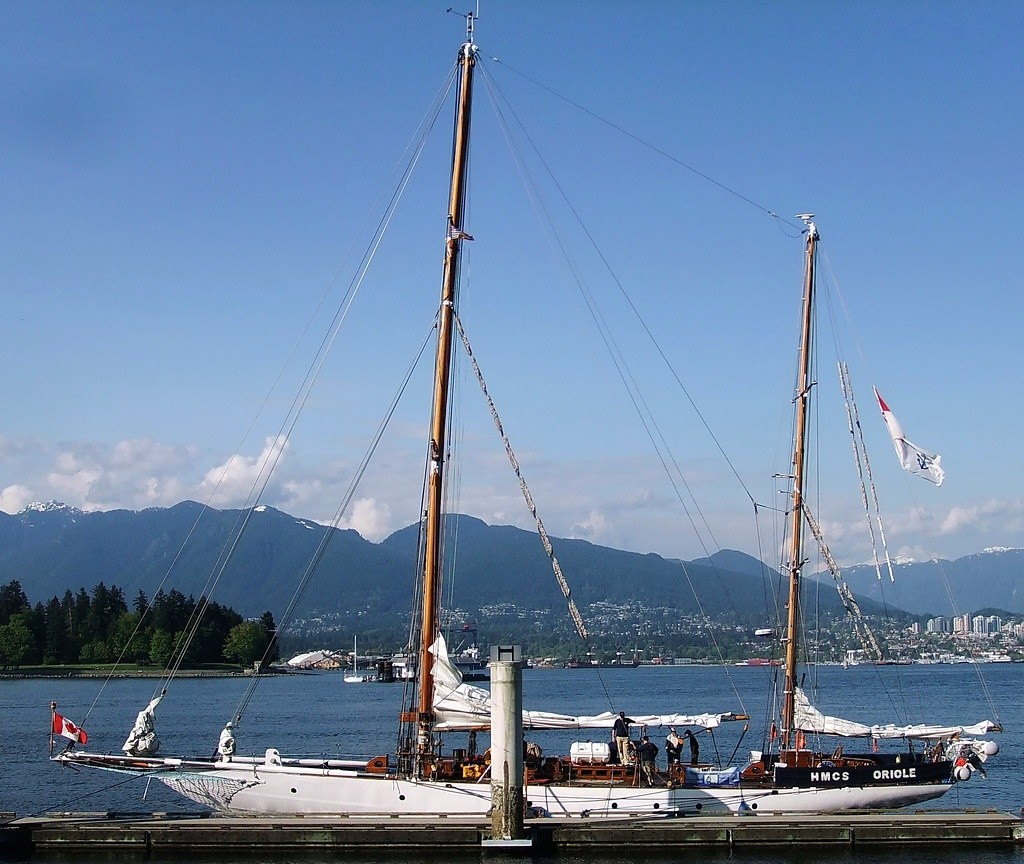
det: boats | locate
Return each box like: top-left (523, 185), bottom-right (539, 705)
top-left (377, 625), bottom-right (492, 684)
top-left (563, 643), bottom-right (641, 668)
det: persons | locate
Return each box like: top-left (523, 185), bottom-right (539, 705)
top-left (685, 729), bottom-right (699, 766)
top-left (630, 737), bottom-right (659, 788)
top-left (611, 711), bottom-right (644, 764)
top-left (521, 733), bottom-right (528, 765)
top-left (666, 728), bottom-right (683, 771)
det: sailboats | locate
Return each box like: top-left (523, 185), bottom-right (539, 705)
top-left (47, 4), bottom-right (1004, 829)
top-left (342, 635), bottom-right (365, 683)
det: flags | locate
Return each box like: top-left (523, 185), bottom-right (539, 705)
top-left (874, 388), bottom-right (945, 485)
top-left (52, 711), bottom-right (89, 744)
